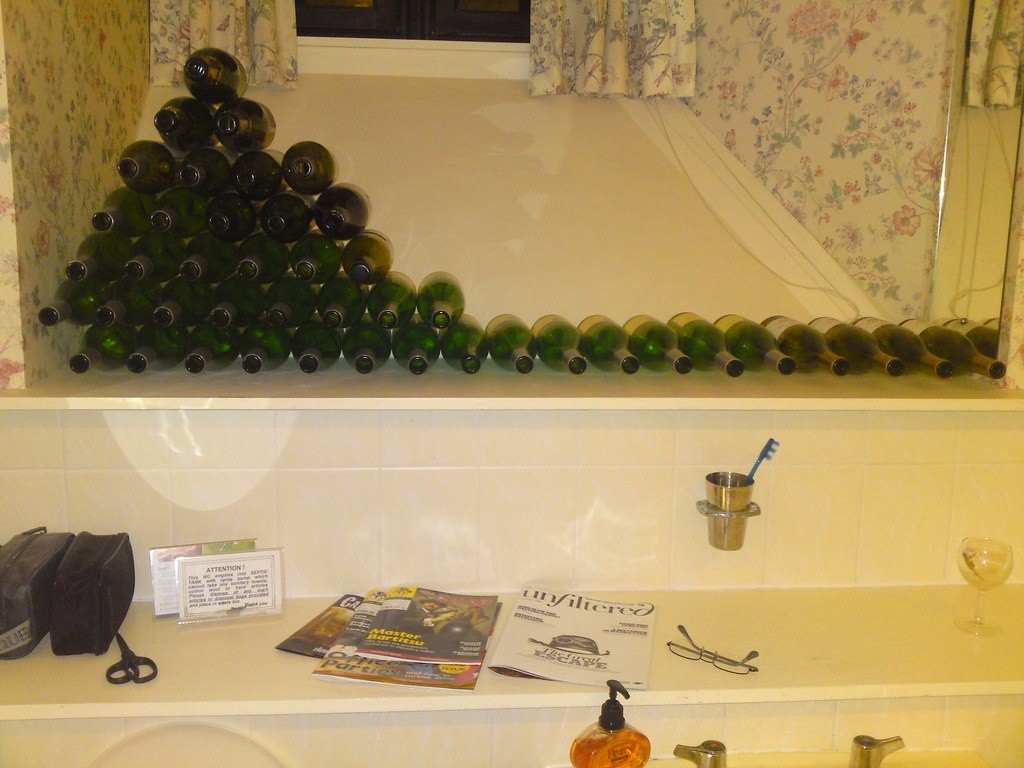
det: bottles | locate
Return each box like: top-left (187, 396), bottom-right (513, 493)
top-left (484, 313), bottom-right (1007, 379)
top-left (39, 49), bottom-right (484, 376)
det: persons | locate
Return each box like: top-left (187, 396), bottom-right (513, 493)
top-left (397, 594), bottom-right (460, 640)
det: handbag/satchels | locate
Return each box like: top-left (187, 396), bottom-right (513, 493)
top-left (50, 531), bottom-right (135, 656)
top-left (0, 526), bottom-right (76, 660)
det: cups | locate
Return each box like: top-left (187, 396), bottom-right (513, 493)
top-left (705, 471), bottom-right (755, 551)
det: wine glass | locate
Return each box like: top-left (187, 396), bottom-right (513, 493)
top-left (952, 537), bottom-right (1013, 634)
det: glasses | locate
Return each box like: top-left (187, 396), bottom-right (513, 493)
top-left (667, 624), bottom-right (758, 675)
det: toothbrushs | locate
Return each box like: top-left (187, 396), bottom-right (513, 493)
top-left (742, 437), bottom-right (780, 486)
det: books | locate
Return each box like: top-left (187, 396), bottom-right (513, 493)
top-left (310, 587), bottom-right (502, 691)
top-left (352, 584), bottom-right (498, 666)
top-left (486, 587), bottom-right (655, 690)
top-left (275, 594), bottom-right (363, 659)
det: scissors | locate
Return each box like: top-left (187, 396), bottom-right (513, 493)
top-left (105, 632), bottom-right (157, 685)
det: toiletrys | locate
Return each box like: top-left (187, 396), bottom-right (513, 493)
top-left (567, 675), bottom-right (651, 768)
